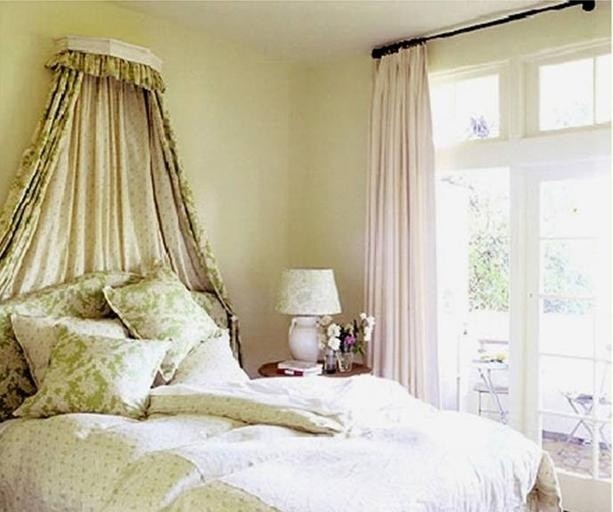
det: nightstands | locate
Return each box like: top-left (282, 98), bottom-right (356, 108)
top-left (258, 360), bottom-right (370, 378)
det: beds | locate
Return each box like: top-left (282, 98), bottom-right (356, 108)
top-left (0, 37), bottom-right (566, 512)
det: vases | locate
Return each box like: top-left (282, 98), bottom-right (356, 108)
top-left (336, 352), bottom-right (352, 372)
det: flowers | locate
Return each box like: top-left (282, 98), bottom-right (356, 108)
top-left (327, 312), bottom-right (376, 354)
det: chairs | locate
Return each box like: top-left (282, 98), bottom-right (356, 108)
top-left (474, 339), bottom-right (517, 415)
top-left (558, 346), bottom-right (611, 469)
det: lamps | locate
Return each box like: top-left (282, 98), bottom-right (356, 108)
top-left (274, 269), bottom-right (342, 362)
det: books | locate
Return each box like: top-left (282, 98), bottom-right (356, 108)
top-left (275, 368), bottom-right (323, 377)
top-left (276, 358), bottom-right (324, 372)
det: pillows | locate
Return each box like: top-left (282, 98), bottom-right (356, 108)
top-left (10, 258), bottom-right (251, 418)
